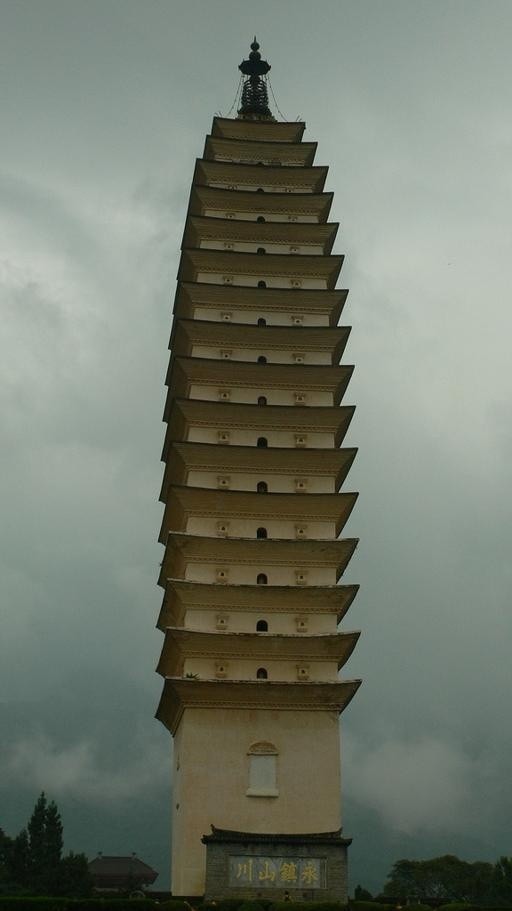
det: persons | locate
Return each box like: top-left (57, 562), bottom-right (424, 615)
top-left (396, 902), bottom-right (402, 909)
top-left (283, 891), bottom-right (292, 903)
